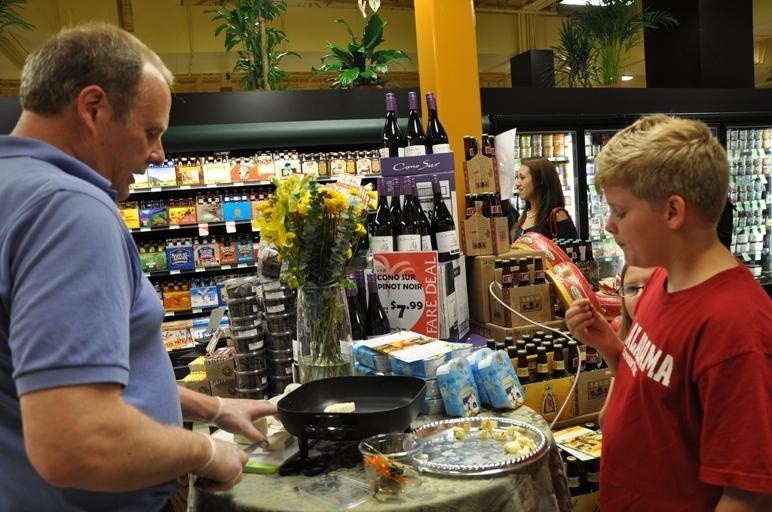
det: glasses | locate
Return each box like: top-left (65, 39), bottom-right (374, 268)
top-left (616, 286), bottom-right (644, 297)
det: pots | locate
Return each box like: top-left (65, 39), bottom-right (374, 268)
top-left (279, 374), bottom-right (428, 440)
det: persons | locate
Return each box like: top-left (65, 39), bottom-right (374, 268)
top-left (507, 155), bottom-right (578, 244)
top-left (598, 261), bottom-right (658, 431)
top-left (0, 23), bottom-right (279, 511)
top-left (564, 111), bottom-right (772, 512)
top-left (502, 200), bottom-right (519, 244)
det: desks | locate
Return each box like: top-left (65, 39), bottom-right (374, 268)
top-left (196, 390), bottom-right (554, 512)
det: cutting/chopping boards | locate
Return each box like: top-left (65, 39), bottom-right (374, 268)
top-left (205, 413), bottom-right (302, 474)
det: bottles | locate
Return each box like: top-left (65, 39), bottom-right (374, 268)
top-left (128, 230), bottom-right (265, 269)
top-left (405, 91), bottom-right (427, 156)
top-left (122, 191), bottom-right (379, 231)
top-left (427, 92), bottom-right (451, 155)
top-left (462, 135), bottom-right (612, 383)
top-left (382, 93), bottom-right (404, 158)
top-left (372, 174), bottom-right (459, 259)
top-left (130, 148), bottom-right (381, 189)
top-left (141, 272), bottom-right (257, 314)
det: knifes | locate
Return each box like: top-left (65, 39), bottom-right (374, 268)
top-left (194, 441), bottom-right (264, 488)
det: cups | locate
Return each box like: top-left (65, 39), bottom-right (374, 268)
top-left (359, 433), bottom-right (423, 495)
